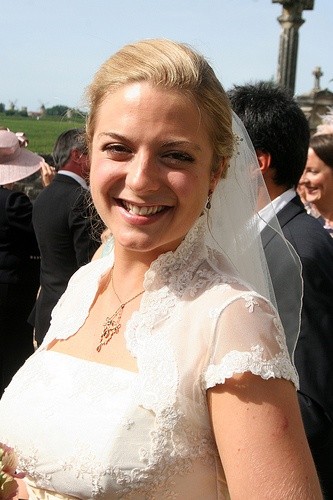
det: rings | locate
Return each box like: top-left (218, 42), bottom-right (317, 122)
top-left (50, 167), bottom-right (56, 172)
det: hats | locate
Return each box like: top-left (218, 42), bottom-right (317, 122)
top-left (0, 127), bottom-right (45, 186)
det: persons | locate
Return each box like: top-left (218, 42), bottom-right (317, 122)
top-left (296, 133), bottom-right (332, 237)
top-left (0, 127), bottom-right (57, 398)
top-left (224, 80), bottom-right (333, 500)
top-left (0, 36), bottom-right (325, 500)
top-left (27, 129), bottom-right (109, 349)
top-left (96, 265), bottom-right (146, 353)
